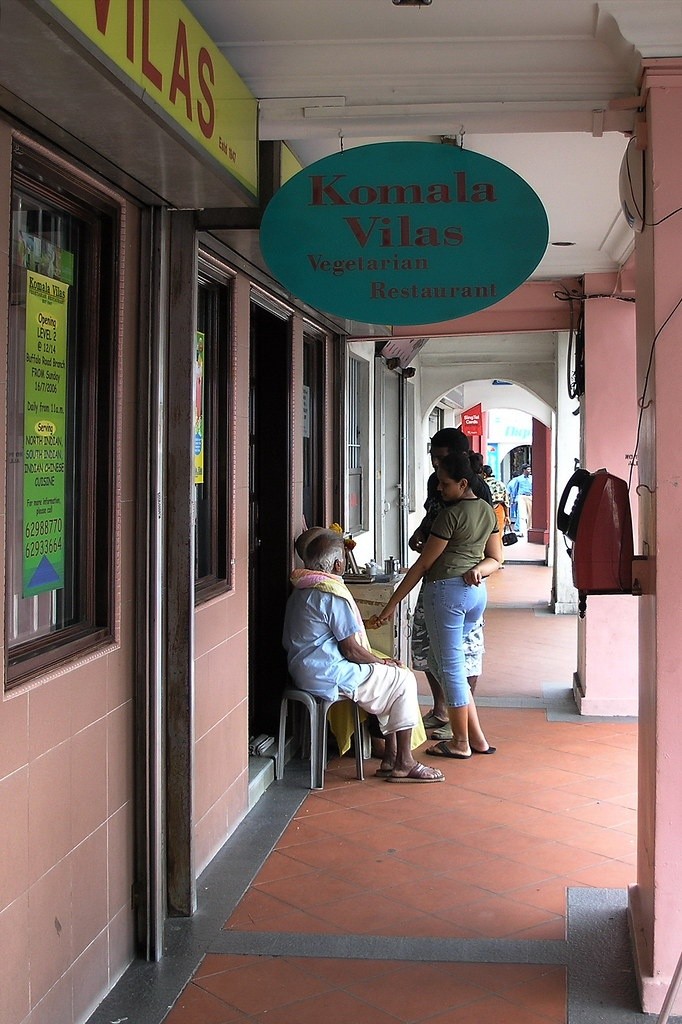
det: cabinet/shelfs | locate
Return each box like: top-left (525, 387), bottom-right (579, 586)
top-left (344, 574), bottom-right (413, 670)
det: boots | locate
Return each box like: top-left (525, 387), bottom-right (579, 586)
top-left (469, 744), bottom-right (496, 754)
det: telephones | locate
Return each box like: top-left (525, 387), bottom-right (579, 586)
top-left (555, 469), bottom-right (635, 596)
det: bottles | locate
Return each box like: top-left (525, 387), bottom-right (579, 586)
top-left (366, 559), bottom-right (377, 576)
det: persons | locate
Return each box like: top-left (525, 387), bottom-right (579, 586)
top-left (288, 527), bottom-right (446, 783)
top-left (479, 464), bottom-right (511, 570)
top-left (505, 463), bottom-right (533, 537)
top-left (408, 425), bottom-right (494, 743)
top-left (370, 452), bottom-right (504, 759)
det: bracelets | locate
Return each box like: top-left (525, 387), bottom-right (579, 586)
top-left (382, 657), bottom-right (388, 666)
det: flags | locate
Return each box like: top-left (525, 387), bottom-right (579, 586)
top-left (461, 403), bottom-right (483, 437)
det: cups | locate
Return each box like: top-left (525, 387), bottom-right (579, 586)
top-left (385, 556), bottom-right (399, 578)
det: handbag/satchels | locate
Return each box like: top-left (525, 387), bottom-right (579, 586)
top-left (502, 532), bottom-right (518, 546)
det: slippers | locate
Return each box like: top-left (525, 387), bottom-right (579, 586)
top-left (375, 761), bottom-right (446, 783)
top-left (425, 741), bottom-right (472, 759)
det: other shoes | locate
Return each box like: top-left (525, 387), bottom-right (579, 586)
top-left (422, 709), bottom-right (448, 729)
top-left (431, 722), bottom-right (454, 740)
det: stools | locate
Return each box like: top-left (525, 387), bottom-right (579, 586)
top-left (276, 689), bottom-right (365, 791)
